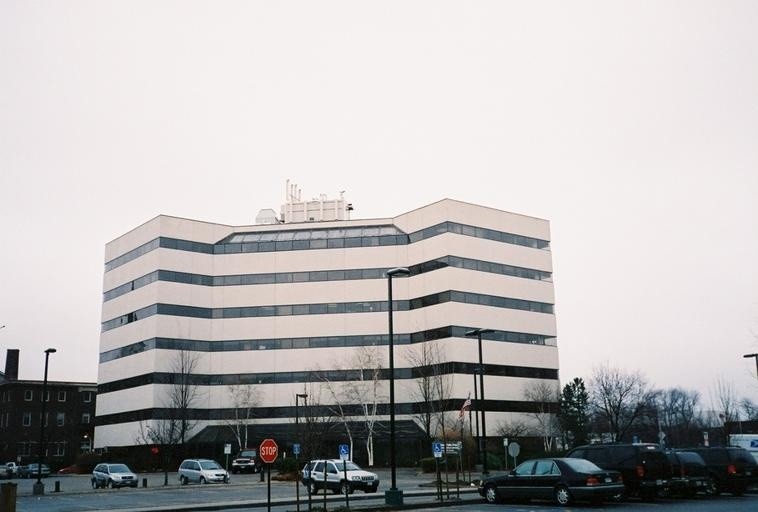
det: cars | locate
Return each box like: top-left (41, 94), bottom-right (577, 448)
top-left (0, 465), bottom-right (11, 480)
top-left (478, 456), bottom-right (625, 507)
top-left (18, 463), bottom-right (51, 479)
top-left (59, 463), bottom-right (81, 474)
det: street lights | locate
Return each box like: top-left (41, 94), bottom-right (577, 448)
top-left (464, 327), bottom-right (498, 479)
top-left (83, 434), bottom-right (92, 452)
top-left (294, 394), bottom-right (308, 444)
top-left (385, 267), bottom-right (410, 490)
top-left (33, 348), bottom-right (56, 484)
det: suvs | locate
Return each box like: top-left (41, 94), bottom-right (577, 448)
top-left (91, 463), bottom-right (139, 489)
top-left (301, 458), bottom-right (380, 494)
top-left (176, 458), bottom-right (230, 485)
top-left (232, 448), bottom-right (262, 474)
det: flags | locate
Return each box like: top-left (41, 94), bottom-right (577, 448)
top-left (459, 394), bottom-right (471, 417)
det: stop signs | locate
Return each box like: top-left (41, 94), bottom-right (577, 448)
top-left (260, 439), bottom-right (279, 463)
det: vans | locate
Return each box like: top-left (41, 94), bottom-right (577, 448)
top-left (564, 443), bottom-right (674, 502)
top-left (665, 445), bottom-right (758, 499)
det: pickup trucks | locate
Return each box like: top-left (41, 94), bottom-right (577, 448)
top-left (6, 462), bottom-right (29, 474)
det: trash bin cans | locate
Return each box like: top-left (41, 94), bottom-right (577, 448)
top-left (0, 482), bottom-right (18, 512)
